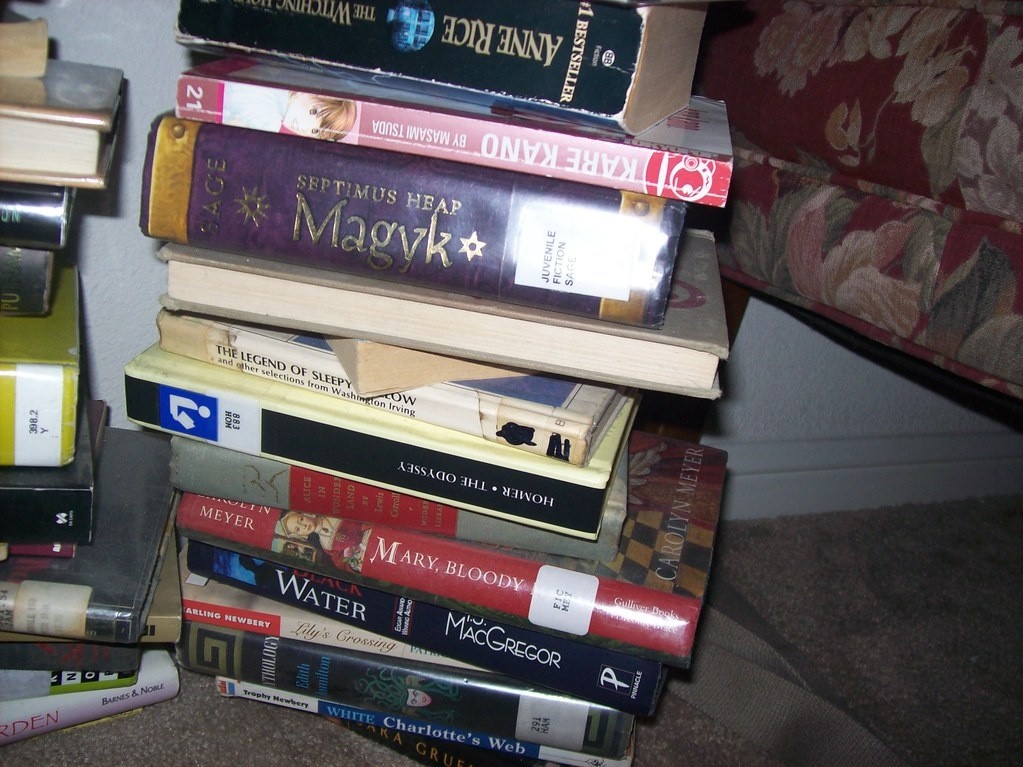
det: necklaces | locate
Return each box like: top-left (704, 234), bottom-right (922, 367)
top-left (317, 518), bottom-right (336, 537)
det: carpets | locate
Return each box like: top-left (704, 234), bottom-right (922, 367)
top-left (703, 490), bottom-right (1023, 767)
top-left (0, 633), bottom-right (912, 767)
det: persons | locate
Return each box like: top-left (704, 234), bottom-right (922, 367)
top-left (223, 84), bottom-right (358, 141)
top-left (281, 511), bottom-right (371, 574)
top-left (0, 0), bottom-right (734, 767)
